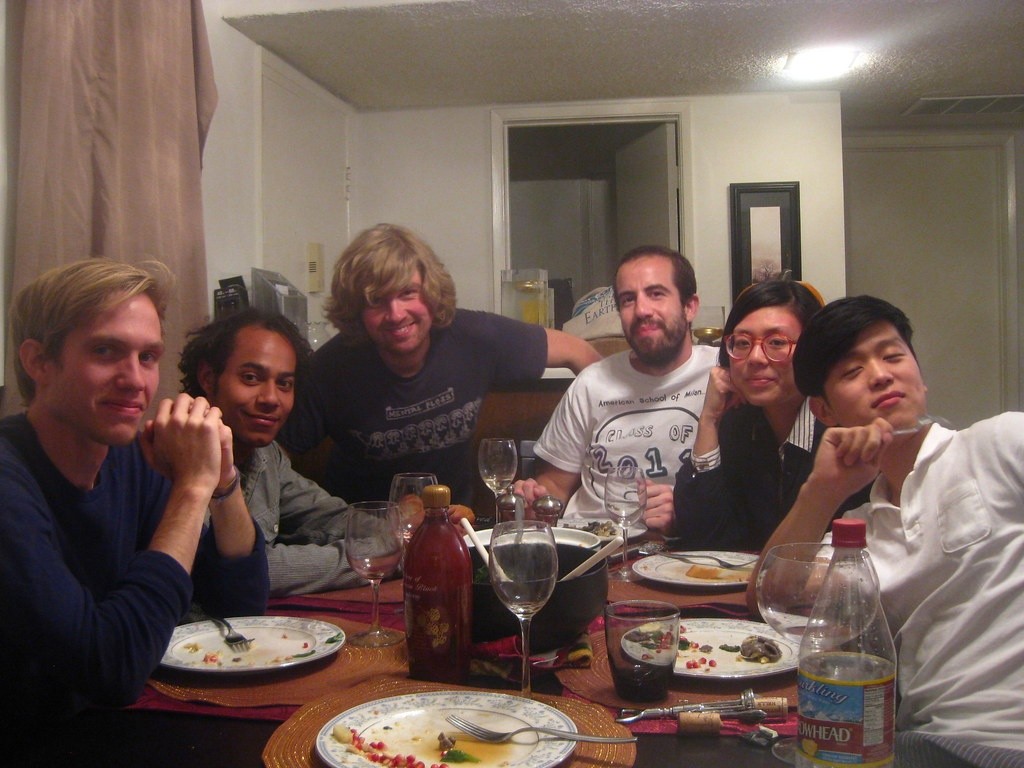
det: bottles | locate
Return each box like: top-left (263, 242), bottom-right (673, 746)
top-left (290, 320), bottom-right (331, 353)
top-left (532, 495), bottom-right (563, 528)
top-left (496, 486), bottom-right (525, 523)
top-left (797, 518), bottom-right (897, 768)
top-left (403, 484), bottom-right (473, 687)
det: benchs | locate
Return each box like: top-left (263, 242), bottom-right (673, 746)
top-left (469, 377), bottom-right (578, 527)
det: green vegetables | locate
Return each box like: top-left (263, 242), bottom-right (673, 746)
top-left (474, 564), bottom-right (515, 584)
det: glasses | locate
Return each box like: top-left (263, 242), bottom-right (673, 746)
top-left (723, 332), bottom-right (796, 362)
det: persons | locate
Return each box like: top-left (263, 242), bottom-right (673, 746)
top-left (0, 258), bottom-right (271, 709)
top-left (178, 307), bottom-right (475, 598)
top-left (746, 294), bottom-right (1024, 767)
top-left (274, 223), bottom-right (604, 519)
top-left (497, 244), bottom-right (722, 542)
top-left (673, 278), bottom-right (882, 552)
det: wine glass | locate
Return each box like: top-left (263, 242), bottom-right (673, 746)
top-left (345, 500), bottom-right (406, 649)
top-left (478, 437), bottom-right (518, 525)
top-left (489, 520), bottom-right (558, 701)
top-left (755, 541), bottom-right (881, 765)
top-left (604, 466), bottom-right (648, 583)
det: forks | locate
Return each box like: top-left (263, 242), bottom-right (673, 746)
top-left (651, 548), bottom-right (759, 570)
top-left (207, 614), bottom-right (254, 645)
top-left (445, 714), bottom-right (639, 744)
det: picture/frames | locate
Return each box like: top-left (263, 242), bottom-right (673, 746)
top-left (730, 182), bottom-right (802, 306)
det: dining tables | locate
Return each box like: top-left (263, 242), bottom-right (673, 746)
top-left (80, 548), bottom-right (794, 768)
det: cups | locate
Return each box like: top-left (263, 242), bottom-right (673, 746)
top-left (604, 599), bottom-right (681, 702)
top-left (388, 471), bottom-right (439, 577)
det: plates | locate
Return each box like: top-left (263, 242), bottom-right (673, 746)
top-left (621, 617), bottom-right (800, 681)
top-left (315, 691), bottom-right (578, 768)
top-left (557, 518), bottom-right (649, 542)
top-left (632, 551), bottom-right (759, 587)
top-left (160, 614), bottom-right (347, 675)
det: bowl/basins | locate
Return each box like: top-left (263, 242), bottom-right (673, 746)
top-left (463, 527), bottom-right (602, 552)
top-left (467, 544), bottom-right (608, 656)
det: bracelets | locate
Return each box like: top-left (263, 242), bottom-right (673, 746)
top-left (211, 465), bottom-right (240, 502)
